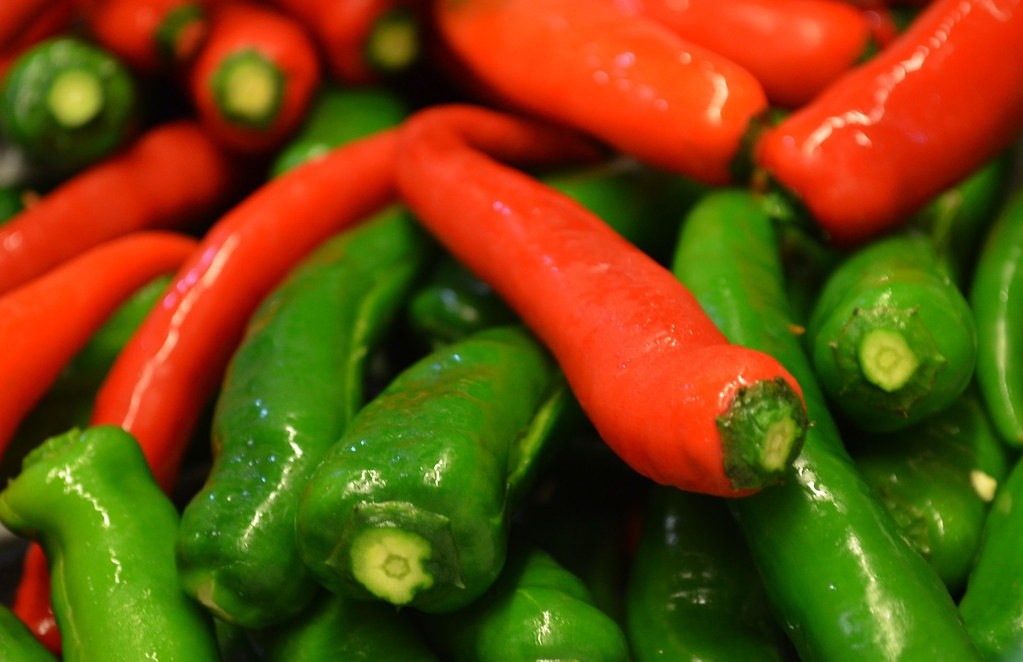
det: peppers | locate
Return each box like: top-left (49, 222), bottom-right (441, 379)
top-left (0, 0), bottom-right (1023, 662)
